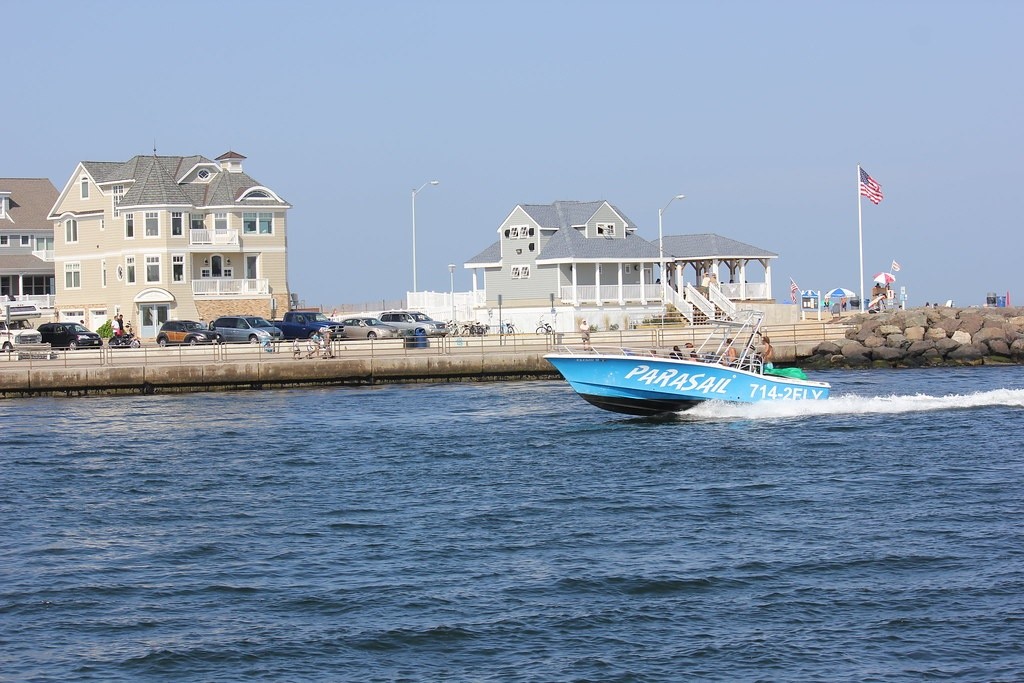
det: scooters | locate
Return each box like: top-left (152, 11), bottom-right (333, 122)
top-left (108, 321), bottom-right (141, 349)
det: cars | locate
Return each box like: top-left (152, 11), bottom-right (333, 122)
top-left (37, 322), bottom-right (104, 351)
top-left (156, 319), bottom-right (219, 346)
top-left (340, 317), bottom-right (399, 340)
top-left (212, 315), bottom-right (284, 345)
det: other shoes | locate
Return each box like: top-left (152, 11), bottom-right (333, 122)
top-left (309, 357), bottom-right (312, 359)
top-left (306, 355), bottom-right (308, 359)
top-left (298, 357), bottom-right (302, 359)
top-left (330, 355), bottom-right (334, 358)
top-left (323, 357), bottom-right (327, 359)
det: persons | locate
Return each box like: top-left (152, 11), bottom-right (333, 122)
top-left (650, 335), bottom-right (774, 376)
top-left (729, 279), bottom-right (734, 283)
top-left (79, 320), bottom-right (86, 332)
top-left (580, 319), bottom-right (593, 351)
top-left (701, 273), bottom-right (718, 300)
top-left (656, 278), bottom-right (660, 284)
top-left (291, 326), bottom-right (334, 360)
top-left (111, 314), bottom-right (124, 338)
top-left (924, 301), bottom-right (939, 307)
top-left (875, 283), bottom-right (890, 297)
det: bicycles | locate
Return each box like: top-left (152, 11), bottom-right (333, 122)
top-left (536, 315), bottom-right (553, 335)
top-left (445, 319), bottom-right (488, 337)
top-left (496, 318), bottom-right (515, 332)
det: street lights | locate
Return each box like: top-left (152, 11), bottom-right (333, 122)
top-left (411, 181), bottom-right (440, 292)
top-left (447, 262), bottom-right (457, 324)
top-left (659, 194), bottom-right (687, 307)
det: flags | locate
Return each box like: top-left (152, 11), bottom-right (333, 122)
top-left (790, 277), bottom-right (797, 305)
top-left (860, 167), bottom-right (883, 205)
top-left (892, 260), bottom-right (901, 272)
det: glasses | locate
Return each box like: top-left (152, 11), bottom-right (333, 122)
top-left (762, 340), bottom-right (766, 341)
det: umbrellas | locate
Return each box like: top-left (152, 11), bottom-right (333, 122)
top-left (825, 288), bottom-right (854, 309)
top-left (871, 271), bottom-right (895, 288)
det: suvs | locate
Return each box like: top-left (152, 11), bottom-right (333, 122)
top-left (378, 310), bottom-right (449, 337)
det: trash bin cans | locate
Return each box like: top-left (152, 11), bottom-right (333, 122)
top-left (415, 327), bottom-right (426, 347)
top-left (403, 329), bottom-right (415, 348)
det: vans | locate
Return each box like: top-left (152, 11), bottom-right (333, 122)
top-left (0, 300), bottom-right (43, 354)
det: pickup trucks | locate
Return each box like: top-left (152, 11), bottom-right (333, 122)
top-left (266, 311), bottom-right (347, 342)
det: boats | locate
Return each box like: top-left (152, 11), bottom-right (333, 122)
top-left (545, 308), bottom-right (832, 420)
